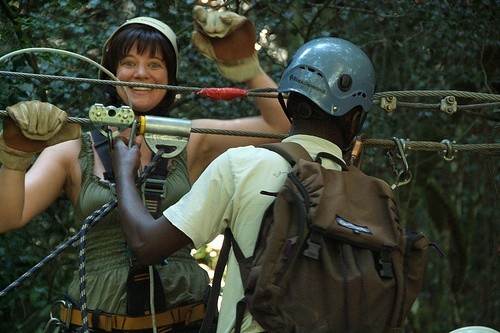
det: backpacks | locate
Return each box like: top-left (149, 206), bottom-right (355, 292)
top-left (231, 141), bottom-right (429, 333)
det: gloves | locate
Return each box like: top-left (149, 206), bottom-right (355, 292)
top-left (0, 100), bottom-right (81, 171)
top-left (190, 6), bottom-right (261, 85)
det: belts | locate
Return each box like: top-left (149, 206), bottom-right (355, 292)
top-left (60, 301), bottom-right (206, 333)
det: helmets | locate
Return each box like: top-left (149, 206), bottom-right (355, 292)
top-left (277, 37), bottom-right (376, 116)
top-left (96, 15), bottom-right (179, 84)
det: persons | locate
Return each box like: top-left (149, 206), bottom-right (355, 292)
top-left (110, 38), bottom-right (377, 333)
top-left (0, 6), bottom-right (294, 333)
